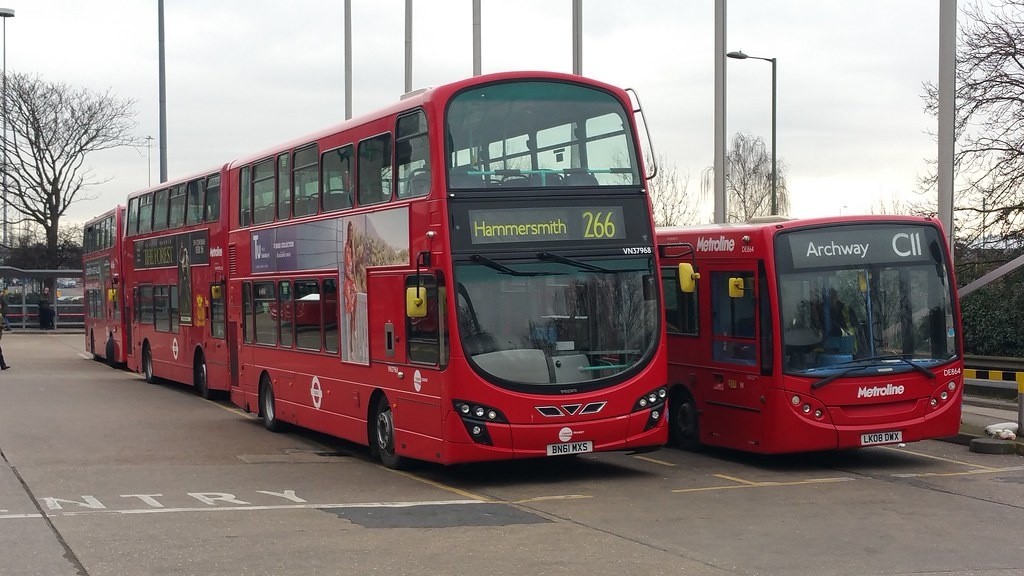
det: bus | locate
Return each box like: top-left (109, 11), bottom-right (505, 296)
top-left (486, 214), bottom-right (968, 469)
top-left (120, 162), bottom-right (236, 402)
top-left (227, 70), bottom-right (702, 480)
top-left (81, 203), bottom-right (131, 368)
top-left (263, 276), bottom-right (344, 328)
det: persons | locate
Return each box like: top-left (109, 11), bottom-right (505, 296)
top-left (0, 287), bottom-right (10, 370)
top-left (818, 288), bottom-right (847, 337)
top-left (343, 220), bottom-right (359, 358)
top-left (37, 286), bottom-right (55, 330)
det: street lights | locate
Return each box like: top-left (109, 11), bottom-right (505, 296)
top-left (0, 7), bottom-right (16, 246)
top-left (726, 50), bottom-right (778, 218)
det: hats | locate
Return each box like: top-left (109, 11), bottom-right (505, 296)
top-left (44, 287), bottom-right (49, 291)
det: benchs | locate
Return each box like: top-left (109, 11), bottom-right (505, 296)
top-left (6, 313), bottom-right (85, 328)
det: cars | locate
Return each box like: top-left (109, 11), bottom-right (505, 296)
top-left (57, 278), bottom-right (77, 289)
top-left (57, 296), bottom-right (76, 303)
top-left (71, 295), bottom-right (85, 304)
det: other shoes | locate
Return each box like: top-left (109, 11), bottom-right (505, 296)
top-left (4, 327), bottom-right (12, 331)
top-left (1, 365), bottom-right (10, 370)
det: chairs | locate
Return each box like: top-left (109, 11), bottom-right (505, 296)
top-left (242, 167), bottom-right (599, 226)
